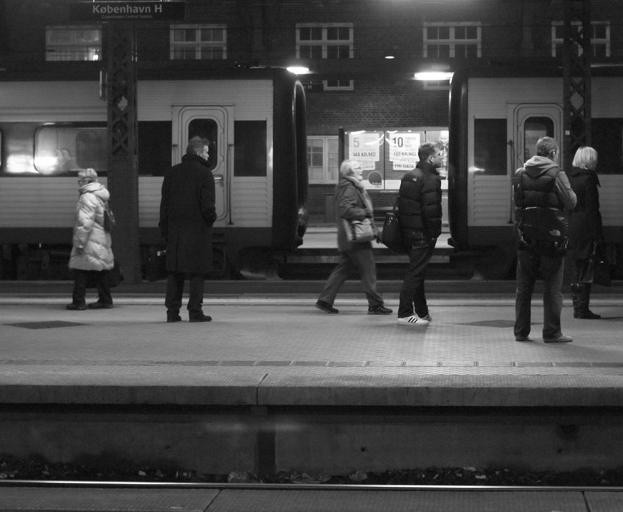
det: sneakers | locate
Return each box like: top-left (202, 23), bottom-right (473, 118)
top-left (365, 304), bottom-right (394, 313)
top-left (397, 313), bottom-right (433, 327)
top-left (188, 309), bottom-right (213, 323)
top-left (542, 334), bottom-right (574, 344)
top-left (166, 311), bottom-right (182, 322)
top-left (513, 331), bottom-right (530, 342)
top-left (315, 300), bottom-right (339, 314)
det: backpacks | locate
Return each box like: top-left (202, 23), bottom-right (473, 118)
top-left (93, 192), bottom-right (119, 234)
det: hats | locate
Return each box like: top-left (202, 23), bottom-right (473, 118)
top-left (77, 168), bottom-right (97, 177)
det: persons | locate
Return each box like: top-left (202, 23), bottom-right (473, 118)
top-left (66, 169), bottom-right (116, 310)
top-left (159, 136), bottom-right (216, 322)
top-left (316, 159), bottom-right (392, 314)
top-left (567, 146), bottom-right (604, 319)
top-left (515, 137), bottom-right (577, 343)
top-left (398, 144), bottom-right (441, 326)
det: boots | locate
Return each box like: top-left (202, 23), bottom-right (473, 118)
top-left (578, 282), bottom-right (602, 319)
top-left (571, 283), bottom-right (580, 319)
top-left (89, 288), bottom-right (116, 308)
top-left (65, 288), bottom-right (85, 311)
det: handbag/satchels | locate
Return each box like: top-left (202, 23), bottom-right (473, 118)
top-left (141, 246), bottom-right (175, 282)
top-left (338, 216), bottom-right (379, 244)
top-left (382, 212), bottom-right (406, 249)
top-left (538, 232), bottom-right (569, 257)
top-left (590, 256), bottom-right (613, 286)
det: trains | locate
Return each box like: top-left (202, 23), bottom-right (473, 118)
top-left (1, 57), bottom-right (623, 280)
